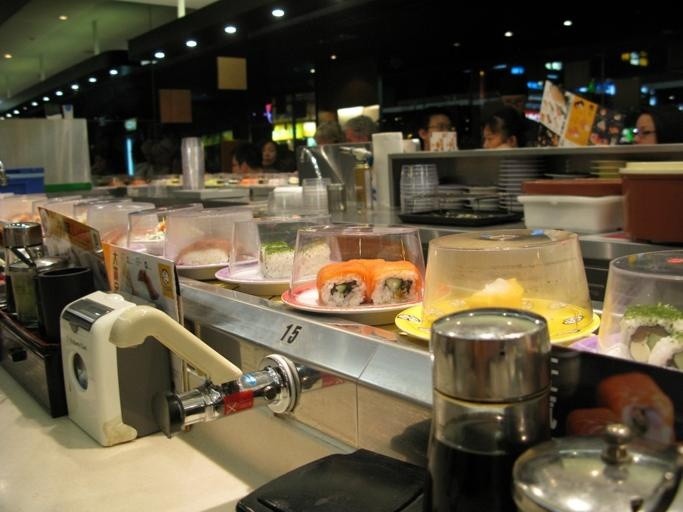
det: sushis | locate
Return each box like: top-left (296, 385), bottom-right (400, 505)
top-left (596, 373), bottom-right (673, 448)
top-left (566, 408), bottom-right (618, 437)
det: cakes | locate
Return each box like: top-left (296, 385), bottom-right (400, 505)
top-left (317, 259), bottom-right (422, 308)
top-left (464, 277), bottom-right (524, 308)
top-left (259, 239), bottom-right (294, 280)
top-left (619, 302), bottom-right (683, 361)
top-left (646, 334), bottom-right (683, 370)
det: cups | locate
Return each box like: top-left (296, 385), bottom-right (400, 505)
top-left (182, 138), bottom-right (205, 189)
top-left (38, 265), bottom-right (95, 342)
top-left (327, 183), bottom-right (346, 214)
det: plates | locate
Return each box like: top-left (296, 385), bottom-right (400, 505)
top-left (282, 281), bottom-right (451, 325)
top-left (394, 298), bottom-right (601, 343)
top-left (440, 158), bottom-right (625, 213)
top-left (133, 249), bottom-right (262, 280)
top-left (213, 263), bottom-right (339, 293)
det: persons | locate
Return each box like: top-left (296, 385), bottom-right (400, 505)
top-left (477, 105), bottom-right (526, 149)
top-left (417, 105), bottom-right (456, 151)
top-left (634, 107), bottom-right (683, 145)
top-left (231, 138), bottom-right (280, 173)
top-left (313, 120), bottom-right (347, 144)
top-left (343, 115), bottom-right (378, 142)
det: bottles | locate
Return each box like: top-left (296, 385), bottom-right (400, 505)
top-left (4, 222), bottom-right (44, 318)
top-left (427, 308), bottom-right (551, 512)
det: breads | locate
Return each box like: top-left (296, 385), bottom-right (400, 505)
top-left (174, 240), bottom-right (248, 265)
